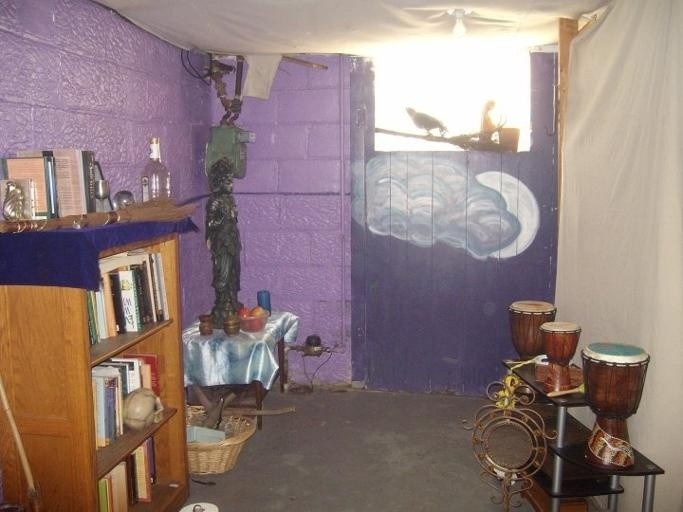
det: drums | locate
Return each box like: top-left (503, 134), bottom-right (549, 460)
top-left (507, 300), bottom-right (557, 360)
top-left (581, 342), bottom-right (650, 472)
top-left (539, 321), bottom-right (582, 393)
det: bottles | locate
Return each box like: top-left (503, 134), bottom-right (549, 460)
top-left (142, 136), bottom-right (171, 204)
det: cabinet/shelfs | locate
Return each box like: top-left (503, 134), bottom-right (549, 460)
top-left (0, 213), bottom-right (190, 512)
top-left (501, 356), bottom-right (664, 512)
top-left (186, 308), bottom-right (297, 430)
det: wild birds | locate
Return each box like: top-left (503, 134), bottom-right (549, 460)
top-left (405, 104), bottom-right (449, 141)
top-left (479, 100), bottom-right (497, 144)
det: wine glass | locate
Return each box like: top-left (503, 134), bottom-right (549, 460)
top-left (95, 180), bottom-right (110, 213)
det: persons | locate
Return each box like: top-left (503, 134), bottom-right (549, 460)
top-left (203, 157), bottom-right (243, 321)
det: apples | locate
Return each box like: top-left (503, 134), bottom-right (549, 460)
top-left (239, 306), bottom-right (265, 331)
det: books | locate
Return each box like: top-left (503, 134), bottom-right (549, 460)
top-left (0, 147), bottom-right (96, 220)
top-left (86, 250), bottom-right (174, 512)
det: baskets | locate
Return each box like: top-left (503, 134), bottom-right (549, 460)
top-left (186, 404), bottom-right (257, 475)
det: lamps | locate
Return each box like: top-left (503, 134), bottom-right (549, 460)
top-left (452, 8), bottom-right (465, 39)
